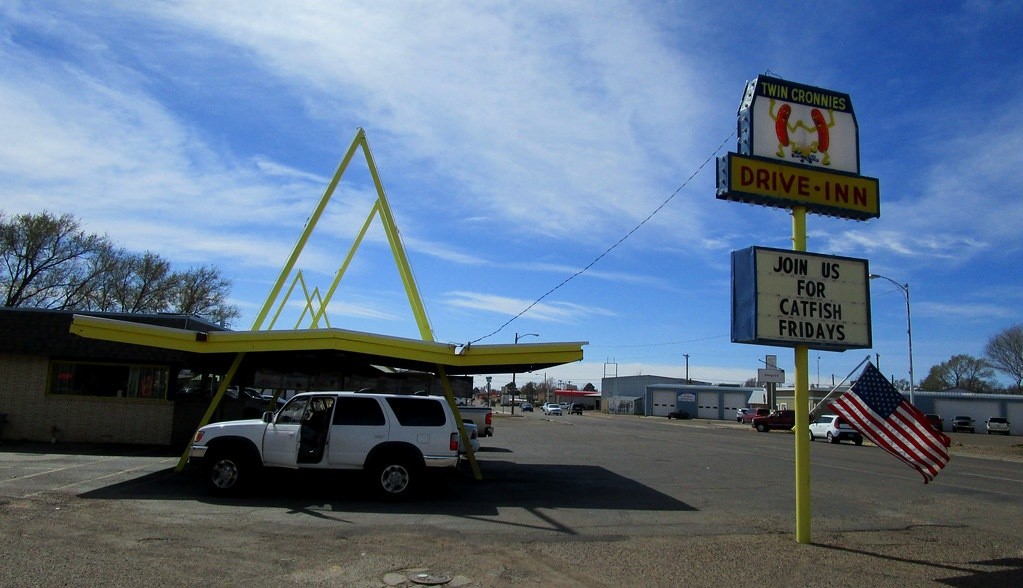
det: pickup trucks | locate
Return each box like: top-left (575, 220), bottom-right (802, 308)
top-left (456, 405), bottom-right (494, 460)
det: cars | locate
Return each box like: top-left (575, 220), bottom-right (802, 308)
top-left (924, 413), bottom-right (944, 432)
top-left (950, 416), bottom-right (975, 433)
top-left (559, 402), bottom-right (570, 409)
top-left (521, 403), bottom-right (533, 412)
top-left (808, 414), bottom-right (863, 446)
top-left (737, 407), bottom-right (769, 424)
top-left (543, 402), bottom-right (562, 415)
top-left (984, 417), bottom-right (1011, 435)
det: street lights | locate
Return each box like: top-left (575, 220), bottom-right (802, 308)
top-left (869, 274), bottom-right (915, 406)
top-left (511, 333), bottom-right (539, 415)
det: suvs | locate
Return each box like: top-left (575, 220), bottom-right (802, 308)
top-left (180, 387), bottom-right (462, 511)
top-left (751, 410), bottom-right (795, 432)
top-left (567, 402), bottom-right (582, 415)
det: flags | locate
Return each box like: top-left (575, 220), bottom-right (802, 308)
top-left (825, 360), bottom-right (952, 485)
